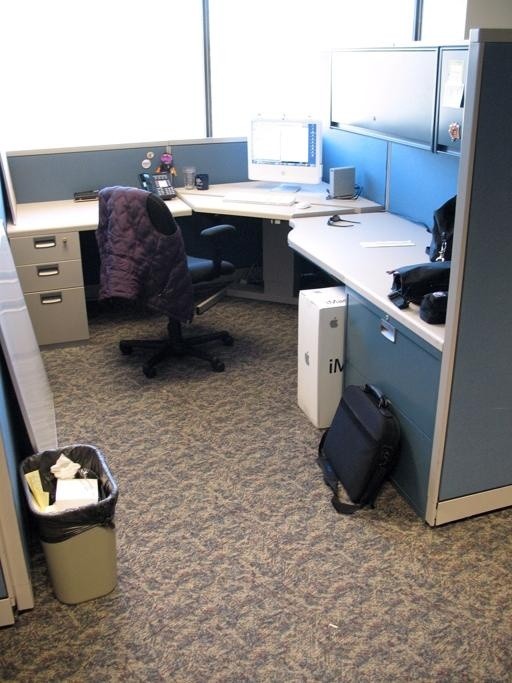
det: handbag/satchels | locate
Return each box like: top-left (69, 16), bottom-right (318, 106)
top-left (426, 195), bottom-right (455, 262)
top-left (315, 384), bottom-right (403, 514)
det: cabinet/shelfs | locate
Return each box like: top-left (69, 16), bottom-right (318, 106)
top-left (341, 287), bottom-right (444, 521)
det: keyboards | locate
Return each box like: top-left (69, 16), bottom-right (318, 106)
top-left (224, 190), bottom-right (298, 208)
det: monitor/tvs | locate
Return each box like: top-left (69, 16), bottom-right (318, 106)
top-left (247, 117), bottom-right (323, 193)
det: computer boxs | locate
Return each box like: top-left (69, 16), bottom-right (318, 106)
top-left (298, 285), bottom-right (346, 429)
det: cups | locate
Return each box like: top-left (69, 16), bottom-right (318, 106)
top-left (181, 165), bottom-right (210, 192)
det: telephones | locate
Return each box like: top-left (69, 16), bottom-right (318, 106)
top-left (137, 172), bottom-right (177, 200)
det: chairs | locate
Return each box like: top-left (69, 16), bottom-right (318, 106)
top-left (94, 188), bottom-right (255, 379)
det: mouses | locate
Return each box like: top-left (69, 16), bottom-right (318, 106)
top-left (298, 201), bottom-right (312, 208)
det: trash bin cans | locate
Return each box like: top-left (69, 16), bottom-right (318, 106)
top-left (19, 443), bottom-right (119, 605)
